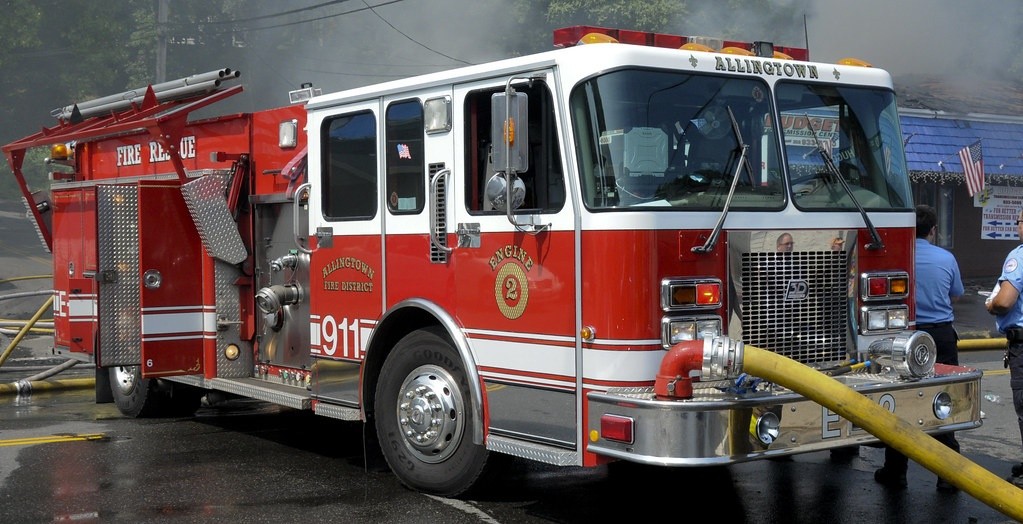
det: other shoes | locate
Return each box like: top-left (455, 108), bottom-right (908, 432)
top-left (874, 468), bottom-right (908, 490)
top-left (1006, 462), bottom-right (1023, 489)
top-left (831, 445), bottom-right (859, 464)
top-left (936, 475), bottom-right (958, 495)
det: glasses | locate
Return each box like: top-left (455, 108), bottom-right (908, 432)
top-left (780, 241), bottom-right (795, 246)
top-left (1016, 220), bottom-right (1023, 225)
top-left (929, 224), bottom-right (938, 231)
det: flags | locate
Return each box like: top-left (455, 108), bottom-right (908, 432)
top-left (958, 139), bottom-right (985, 197)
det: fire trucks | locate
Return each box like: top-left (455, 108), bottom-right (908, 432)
top-left (1, 25), bottom-right (983, 499)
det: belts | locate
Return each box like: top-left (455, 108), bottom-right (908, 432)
top-left (917, 322), bottom-right (951, 329)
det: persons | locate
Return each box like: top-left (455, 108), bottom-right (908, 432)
top-left (874, 205), bottom-right (964, 492)
top-left (832, 237), bottom-right (845, 250)
top-left (985, 209), bottom-right (1023, 476)
top-left (776, 234), bottom-right (793, 252)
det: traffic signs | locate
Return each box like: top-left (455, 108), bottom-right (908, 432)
top-left (981, 183), bottom-right (1023, 241)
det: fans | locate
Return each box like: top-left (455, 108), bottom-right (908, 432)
top-left (698, 107), bottom-right (731, 141)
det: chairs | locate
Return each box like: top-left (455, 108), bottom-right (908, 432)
top-left (688, 137), bottom-right (743, 181)
